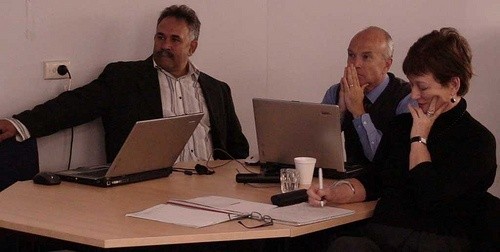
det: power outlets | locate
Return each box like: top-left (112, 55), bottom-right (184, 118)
top-left (43, 61), bottom-right (71, 79)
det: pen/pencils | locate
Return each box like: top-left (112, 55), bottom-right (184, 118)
top-left (319, 168), bottom-right (325, 210)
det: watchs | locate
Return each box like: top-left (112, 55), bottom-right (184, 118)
top-left (409, 135), bottom-right (428, 144)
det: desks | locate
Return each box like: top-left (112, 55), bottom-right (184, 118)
top-left (0, 159), bottom-right (377, 252)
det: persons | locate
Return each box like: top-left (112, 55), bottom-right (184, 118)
top-left (306, 28), bottom-right (499, 252)
top-left (322, 25), bottom-right (418, 166)
top-left (0, 5), bottom-right (250, 164)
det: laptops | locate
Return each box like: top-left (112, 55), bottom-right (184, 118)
top-left (252, 97), bottom-right (366, 180)
top-left (53, 112), bottom-right (205, 187)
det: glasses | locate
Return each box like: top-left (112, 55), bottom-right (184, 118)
top-left (228, 211), bottom-right (274, 229)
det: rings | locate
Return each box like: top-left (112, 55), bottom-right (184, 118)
top-left (427, 111), bottom-right (435, 116)
top-left (348, 84), bottom-right (354, 87)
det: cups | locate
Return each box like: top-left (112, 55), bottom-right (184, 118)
top-left (294, 157), bottom-right (317, 190)
top-left (279, 168), bottom-right (300, 193)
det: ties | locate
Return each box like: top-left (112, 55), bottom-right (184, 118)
top-left (363, 98), bottom-right (371, 113)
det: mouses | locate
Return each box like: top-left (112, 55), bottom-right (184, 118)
top-left (33, 171), bottom-right (61, 185)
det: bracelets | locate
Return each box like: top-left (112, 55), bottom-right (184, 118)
top-left (335, 179), bottom-right (356, 194)
